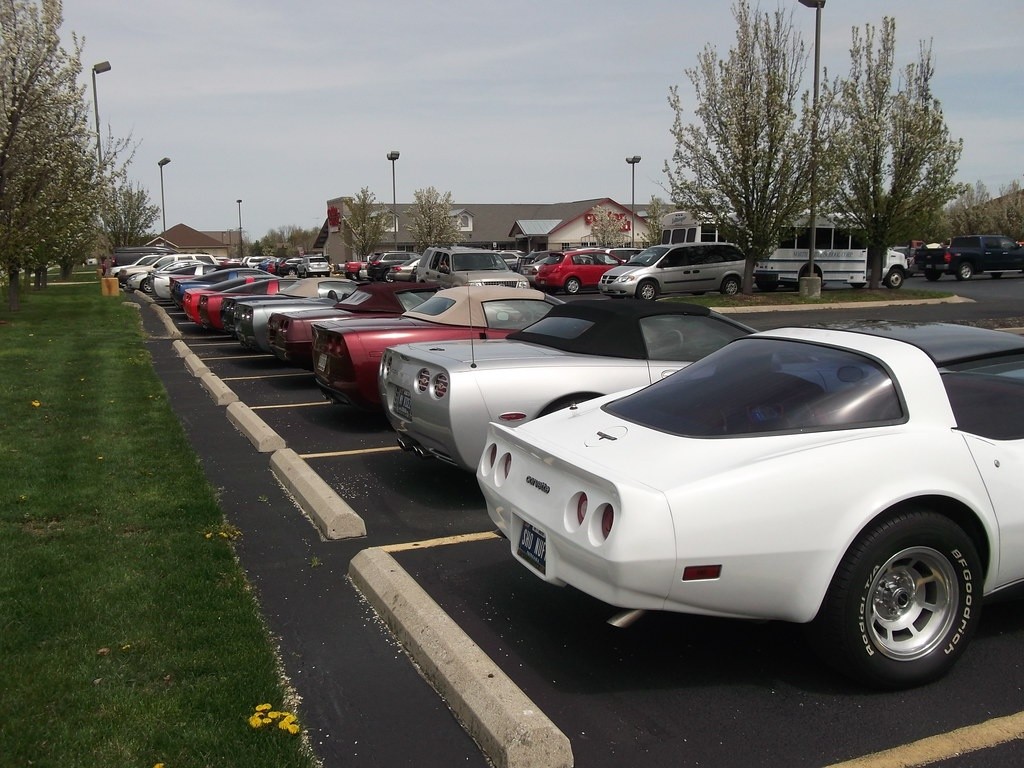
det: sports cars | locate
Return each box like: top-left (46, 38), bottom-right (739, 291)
top-left (474, 318), bottom-right (1024, 687)
top-left (309, 283), bottom-right (566, 413)
top-left (107, 245), bottom-right (634, 349)
top-left (265, 279), bottom-right (452, 374)
top-left (378, 300), bottom-right (763, 478)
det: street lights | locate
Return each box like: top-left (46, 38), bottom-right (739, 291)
top-left (385, 150), bottom-right (399, 252)
top-left (236, 199), bottom-right (244, 258)
top-left (156, 157), bottom-right (170, 250)
top-left (625, 155), bottom-right (642, 249)
top-left (91, 60), bottom-right (116, 278)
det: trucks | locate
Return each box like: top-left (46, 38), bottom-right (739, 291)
top-left (723, 209), bottom-right (909, 291)
top-left (659, 209), bottom-right (729, 246)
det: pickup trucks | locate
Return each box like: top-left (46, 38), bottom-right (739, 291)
top-left (914, 233), bottom-right (1024, 281)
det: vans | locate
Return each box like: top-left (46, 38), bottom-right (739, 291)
top-left (597, 241), bottom-right (756, 301)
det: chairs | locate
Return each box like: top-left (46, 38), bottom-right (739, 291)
top-left (739, 369), bottom-right (822, 419)
